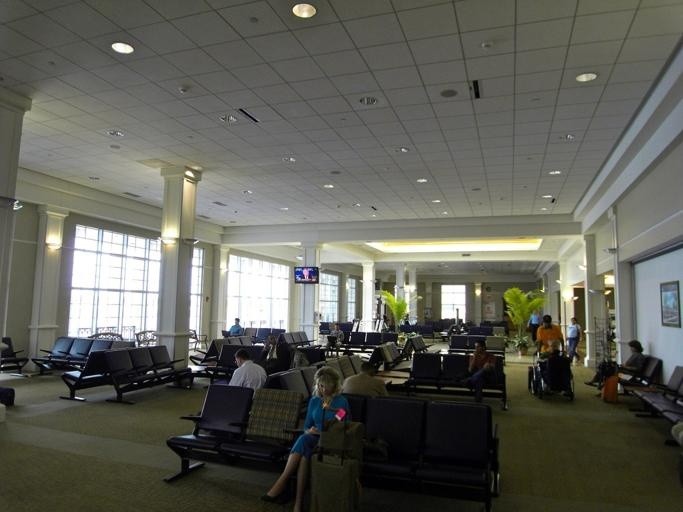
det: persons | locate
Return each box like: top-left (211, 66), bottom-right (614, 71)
top-left (229, 318), bottom-right (241, 334)
top-left (323, 322), bottom-right (345, 360)
top-left (344, 361), bottom-right (390, 397)
top-left (259, 334), bottom-right (292, 375)
top-left (463, 340), bottom-right (496, 401)
top-left (525, 307), bottom-right (644, 398)
top-left (227, 350), bottom-right (268, 399)
top-left (258, 365), bottom-right (351, 511)
top-left (302, 268), bottom-right (313, 279)
top-left (377, 314), bottom-right (388, 331)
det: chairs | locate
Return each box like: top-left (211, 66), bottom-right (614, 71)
top-left (0, 329), bottom-right (191, 410)
top-left (612, 354), bottom-right (682, 438)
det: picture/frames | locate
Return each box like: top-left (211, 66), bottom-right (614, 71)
top-left (659, 280), bottom-right (680, 329)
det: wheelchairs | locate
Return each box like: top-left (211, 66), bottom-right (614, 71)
top-left (527, 350), bottom-right (575, 403)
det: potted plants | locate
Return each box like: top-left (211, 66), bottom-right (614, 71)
top-left (500, 286), bottom-right (544, 361)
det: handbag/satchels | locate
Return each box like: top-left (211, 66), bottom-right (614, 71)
top-left (312, 419), bottom-right (365, 456)
top-left (599, 366), bottom-right (614, 376)
top-left (291, 350), bottom-right (310, 368)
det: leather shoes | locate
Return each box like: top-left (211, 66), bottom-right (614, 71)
top-left (261, 487), bottom-right (284, 501)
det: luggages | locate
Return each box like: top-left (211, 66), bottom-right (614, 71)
top-left (310, 408), bottom-right (362, 512)
top-left (601, 363), bottom-right (617, 402)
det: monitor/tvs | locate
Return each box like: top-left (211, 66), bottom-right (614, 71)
top-left (294, 266), bottom-right (318, 284)
